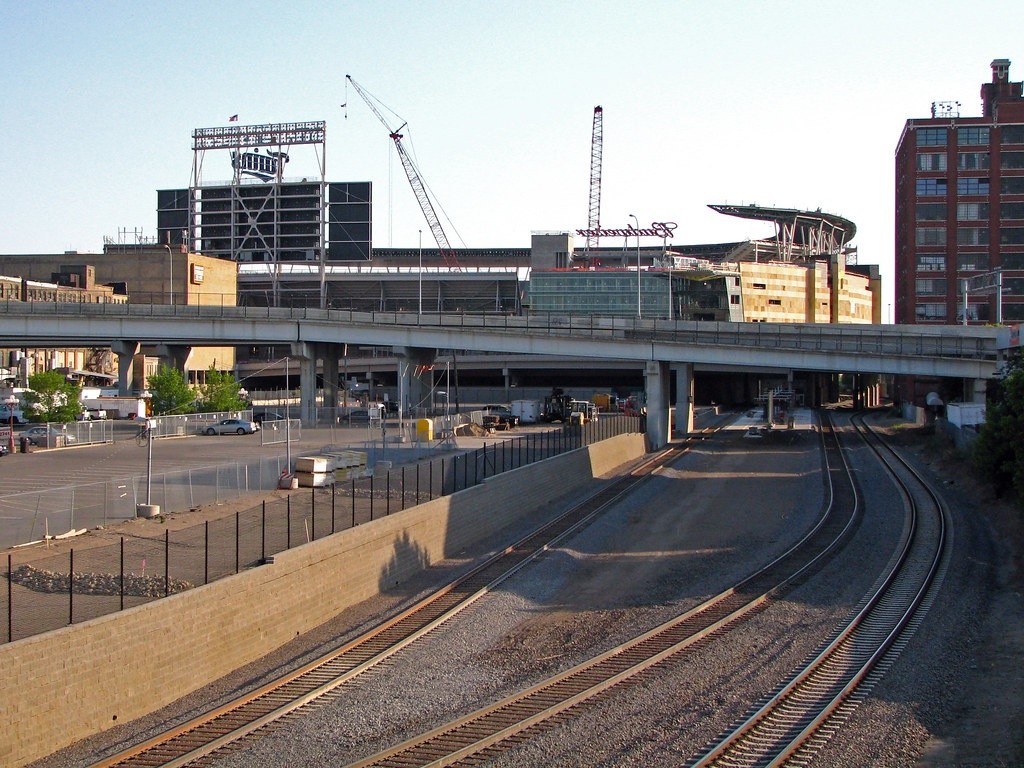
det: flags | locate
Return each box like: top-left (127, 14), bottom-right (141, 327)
top-left (230, 114), bottom-right (239, 121)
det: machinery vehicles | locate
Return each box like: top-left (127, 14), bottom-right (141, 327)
top-left (543, 385), bottom-right (638, 428)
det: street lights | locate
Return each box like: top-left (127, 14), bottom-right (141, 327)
top-left (419, 228), bottom-right (422, 318)
top-left (629, 213), bottom-right (642, 318)
top-left (4, 377), bottom-right (24, 455)
top-left (155, 242), bottom-right (173, 305)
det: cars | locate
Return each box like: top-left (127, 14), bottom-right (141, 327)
top-left (128, 413), bottom-right (136, 420)
top-left (252, 411), bottom-right (285, 424)
top-left (338, 410), bottom-right (371, 424)
top-left (17, 426), bottom-right (75, 447)
top-left (200, 419), bottom-right (257, 435)
top-left (383, 401), bottom-right (400, 409)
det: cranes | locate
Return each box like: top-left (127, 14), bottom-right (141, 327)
top-left (344, 74), bottom-right (467, 275)
